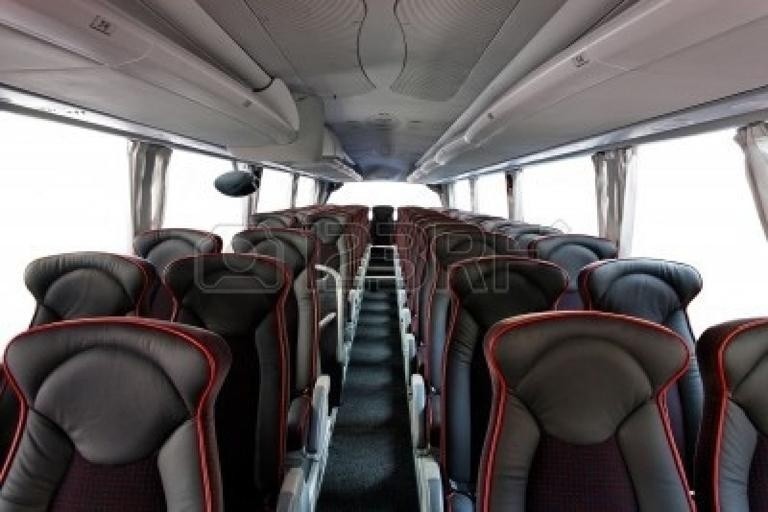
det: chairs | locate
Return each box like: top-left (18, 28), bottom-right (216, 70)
top-left (368, 203), bottom-right (394, 249)
